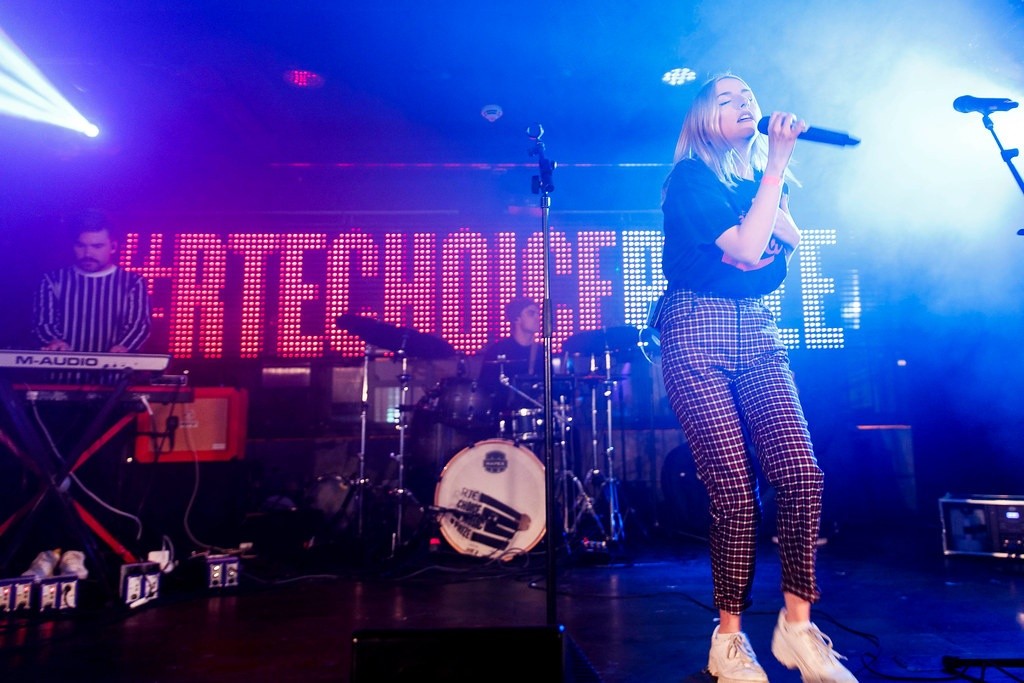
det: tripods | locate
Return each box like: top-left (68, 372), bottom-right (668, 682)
top-left (552, 342), bottom-right (652, 562)
top-left (330, 336), bottom-right (433, 576)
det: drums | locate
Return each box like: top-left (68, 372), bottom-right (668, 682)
top-left (430, 374), bottom-right (502, 435)
top-left (432, 437), bottom-right (549, 562)
top-left (504, 404), bottom-right (574, 444)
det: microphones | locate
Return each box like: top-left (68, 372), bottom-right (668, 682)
top-left (953, 95), bottom-right (1018, 113)
top-left (757, 116), bottom-right (860, 147)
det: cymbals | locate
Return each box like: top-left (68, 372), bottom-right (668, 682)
top-left (560, 325), bottom-right (640, 355)
top-left (480, 356), bottom-right (530, 365)
top-left (333, 313), bottom-right (456, 361)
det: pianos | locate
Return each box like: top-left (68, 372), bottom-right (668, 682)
top-left (0, 348), bottom-right (197, 405)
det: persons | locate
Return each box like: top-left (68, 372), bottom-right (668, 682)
top-left (652, 73), bottom-right (859, 682)
top-left (477, 294), bottom-right (555, 462)
top-left (20, 218), bottom-right (155, 588)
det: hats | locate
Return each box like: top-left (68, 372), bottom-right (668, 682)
top-left (505, 297), bottom-right (540, 321)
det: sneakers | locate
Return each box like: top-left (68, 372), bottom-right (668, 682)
top-left (771, 607), bottom-right (859, 683)
top-left (21, 551), bottom-right (88, 579)
top-left (707, 625), bottom-right (769, 683)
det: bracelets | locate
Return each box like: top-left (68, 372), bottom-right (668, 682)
top-left (760, 174), bottom-right (783, 188)
